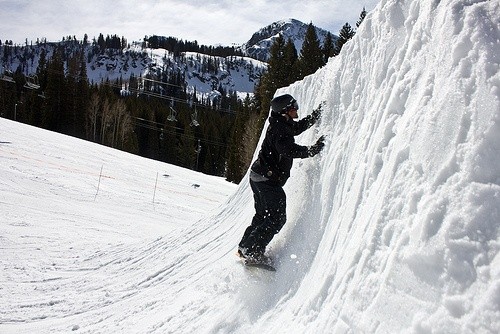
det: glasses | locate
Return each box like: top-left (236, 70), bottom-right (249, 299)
top-left (285, 101), bottom-right (299, 114)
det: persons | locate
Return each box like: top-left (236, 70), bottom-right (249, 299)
top-left (235, 93), bottom-right (327, 271)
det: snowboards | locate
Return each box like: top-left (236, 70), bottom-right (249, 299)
top-left (234, 252), bottom-right (276, 272)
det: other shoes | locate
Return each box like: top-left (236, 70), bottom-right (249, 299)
top-left (237, 243), bottom-right (272, 266)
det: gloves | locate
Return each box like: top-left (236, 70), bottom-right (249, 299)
top-left (308, 134), bottom-right (329, 157)
top-left (304, 100), bottom-right (326, 128)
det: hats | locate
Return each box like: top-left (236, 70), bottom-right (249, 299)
top-left (270, 94), bottom-right (299, 114)
